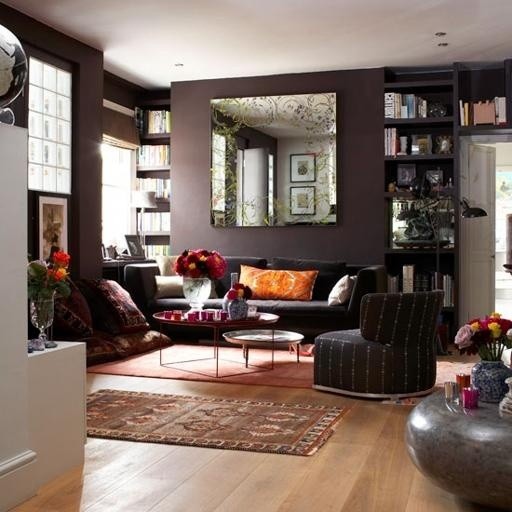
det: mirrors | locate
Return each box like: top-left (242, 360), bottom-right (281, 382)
top-left (209, 93), bottom-right (339, 229)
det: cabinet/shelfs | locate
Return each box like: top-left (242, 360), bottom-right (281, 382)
top-left (383, 59), bottom-right (512, 343)
top-left (136, 87), bottom-right (171, 262)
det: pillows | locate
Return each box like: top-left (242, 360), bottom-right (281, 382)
top-left (239, 265), bottom-right (359, 306)
top-left (58, 278), bottom-right (151, 368)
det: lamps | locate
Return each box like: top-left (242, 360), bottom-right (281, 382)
top-left (416, 194), bottom-right (489, 356)
top-left (133, 192), bottom-right (158, 258)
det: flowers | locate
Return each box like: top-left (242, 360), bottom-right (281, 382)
top-left (452, 314), bottom-right (512, 361)
top-left (174, 248), bottom-right (227, 278)
top-left (227, 281), bottom-right (253, 302)
top-left (28, 250), bottom-right (73, 328)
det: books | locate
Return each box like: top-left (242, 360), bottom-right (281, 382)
top-left (384, 92), bottom-right (427, 157)
top-left (387, 262), bottom-right (452, 307)
top-left (458, 96), bottom-right (506, 126)
top-left (136, 109), bottom-right (172, 257)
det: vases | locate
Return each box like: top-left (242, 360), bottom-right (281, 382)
top-left (29, 289), bottom-right (57, 348)
top-left (228, 300), bottom-right (248, 320)
top-left (470, 359), bottom-right (511, 403)
top-left (181, 277), bottom-right (212, 311)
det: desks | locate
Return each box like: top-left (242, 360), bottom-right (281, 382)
top-left (403, 381), bottom-right (511, 512)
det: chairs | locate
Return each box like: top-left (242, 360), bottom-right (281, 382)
top-left (312, 291), bottom-right (446, 399)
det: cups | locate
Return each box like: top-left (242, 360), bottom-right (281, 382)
top-left (162, 308), bottom-right (228, 322)
top-left (247, 305), bottom-right (257, 318)
top-left (444, 373), bottom-right (478, 410)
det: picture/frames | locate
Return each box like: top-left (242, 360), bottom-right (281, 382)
top-left (39, 196), bottom-right (69, 263)
top-left (289, 153), bottom-right (317, 215)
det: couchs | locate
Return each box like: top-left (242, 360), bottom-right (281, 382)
top-left (123, 255), bottom-right (385, 342)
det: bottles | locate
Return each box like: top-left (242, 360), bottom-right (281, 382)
top-left (222, 273), bottom-right (239, 310)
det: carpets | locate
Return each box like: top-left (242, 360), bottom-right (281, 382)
top-left (88, 343), bottom-right (355, 456)
